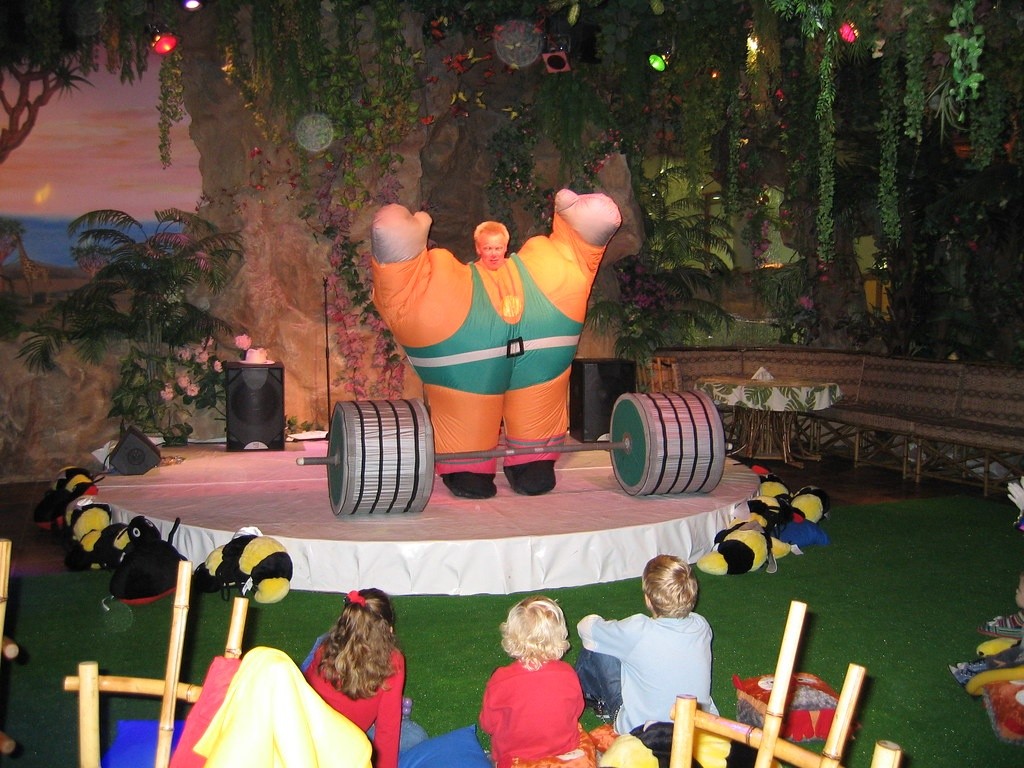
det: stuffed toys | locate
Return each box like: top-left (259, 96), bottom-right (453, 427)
top-left (696, 464), bottom-right (830, 575)
top-left (30, 465), bottom-right (293, 605)
top-left (597, 721), bottom-right (780, 768)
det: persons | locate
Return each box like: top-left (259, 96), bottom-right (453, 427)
top-left (576, 556), bottom-right (720, 735)
top-left (373, 189), bottom-right (621, 499)
top-left (479, 597), bottom-right (585, 768)
top-left (301, 587), bottom-right (405, 768)
top-left (949, 475), bottom-right (1024, 689)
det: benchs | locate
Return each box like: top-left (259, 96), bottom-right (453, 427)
top-left (634, 348), bottom-right (1024, 497)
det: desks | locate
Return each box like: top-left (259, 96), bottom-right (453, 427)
top-left (693, 376), bottom-right (845, 468)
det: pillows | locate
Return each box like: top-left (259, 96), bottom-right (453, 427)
top-left (396, 723), bottom-right (493, 768)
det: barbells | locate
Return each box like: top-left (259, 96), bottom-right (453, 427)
top-left (295, 388), bottom-right (735, 525)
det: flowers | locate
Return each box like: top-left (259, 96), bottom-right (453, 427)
top-left (158, 334), bottom-right (271, 422)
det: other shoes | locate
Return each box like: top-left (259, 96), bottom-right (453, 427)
top-left (977, 616), bottom-right (1023, 639)
top-left (583, 694), bottom-right (611, 719)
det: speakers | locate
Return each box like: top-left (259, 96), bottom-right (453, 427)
top-left (108, 424), bottom-right (162, 475)
top-left (568, 358), bottom-right (637, 443)
top-left (225, 360), bottom-right (284, 452)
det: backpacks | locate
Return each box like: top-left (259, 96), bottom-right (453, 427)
top-left (108, 542), bottom-right (187, 605)
top-left (32, 480), bottom-right (99, 529)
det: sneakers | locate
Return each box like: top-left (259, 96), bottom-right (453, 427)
top-left (947, 665), bottom-right (978, 687)
top-left (956, 658), bottom-right (990, 673)
